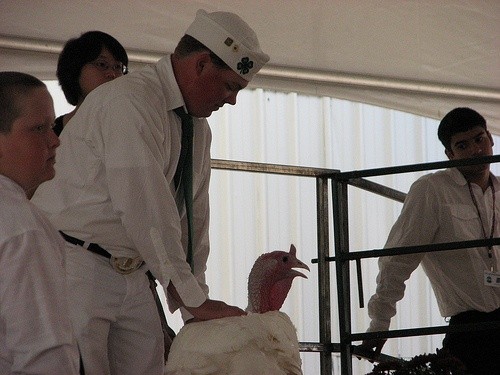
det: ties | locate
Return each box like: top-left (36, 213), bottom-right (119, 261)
top-left (172, 106), bottom-right (196, 275)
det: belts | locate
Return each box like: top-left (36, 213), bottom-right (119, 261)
top-left (58, 229), bottom-right (145, 274)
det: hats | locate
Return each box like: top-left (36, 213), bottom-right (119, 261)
top-left (185, 9), bottom-right (271, 82)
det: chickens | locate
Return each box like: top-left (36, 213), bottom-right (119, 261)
top-left (166, 244), bottom-right (310, 375)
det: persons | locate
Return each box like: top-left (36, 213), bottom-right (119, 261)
top-left (54, 30), bottom-right (128, 133)
top-left (25, 7), bottom-right (270, 373)
top-left (0, 71), bottom-right (69, 375)
top-left (359, 107), bottom-right (499, 375)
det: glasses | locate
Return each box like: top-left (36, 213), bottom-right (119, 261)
top-left (86, 59), bottom-right (127, 74)
